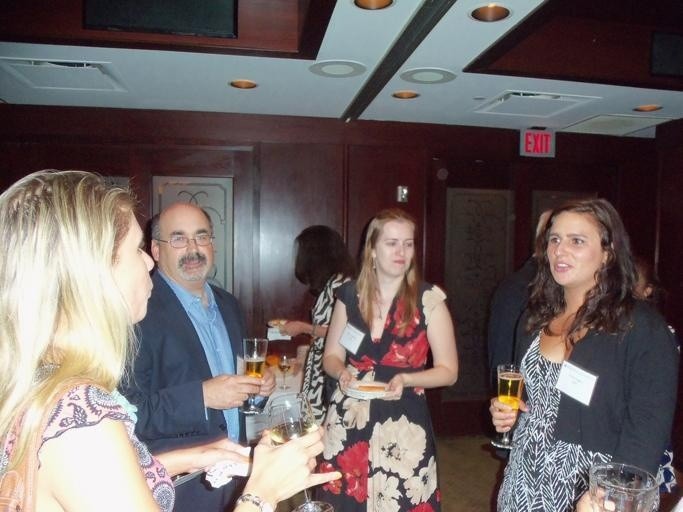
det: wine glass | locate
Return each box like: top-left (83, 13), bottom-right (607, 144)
top-left (239, 338), bottom-right (268, 415)
top-left (491, 363), bottom-right (527, 449)
top-left (278, 354), bottom-right (291, 389)
top-left (268, 392), bottom-right (335, 512)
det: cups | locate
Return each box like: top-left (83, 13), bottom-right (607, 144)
top-left (588, 463), bottom-right (661, 512)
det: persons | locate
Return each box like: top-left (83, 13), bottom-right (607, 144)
top-left (265, 224), bottom-right (352, 429)
top-left (485, 205), bottom-right (554, 462)
top-left (0, 169), bottom-right (342, 512)
top-left (485, 198), bottom-right (681, 510)
top-left (627, 252), bottom-right (680, 495)
top-left (321, 208), bottom-right (461, 511)
top-left (118, 200), bottom-right (276, 512)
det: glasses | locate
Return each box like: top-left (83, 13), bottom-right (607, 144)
top-left (155, 233), bottom-right (215, 248)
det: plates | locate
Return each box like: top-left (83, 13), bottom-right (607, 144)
top-left (345, 380), bottom-right (391, 400)
top-left (266, 323), bottom-right (288, 329)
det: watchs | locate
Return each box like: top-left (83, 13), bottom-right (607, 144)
top-left (229, 493), bottom-right (273, 512)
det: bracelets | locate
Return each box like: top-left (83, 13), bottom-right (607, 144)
top-left (311, 325), bottom-right (318, 335)
